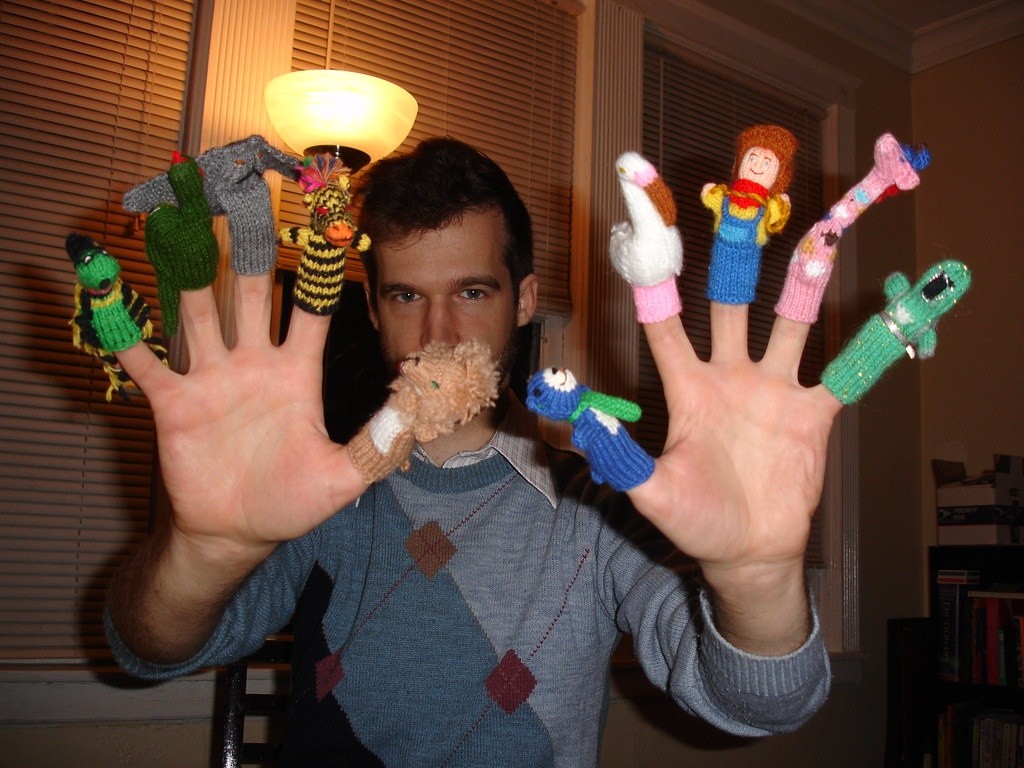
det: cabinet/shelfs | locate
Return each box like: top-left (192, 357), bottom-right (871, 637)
top-left (884, 544), bottom-right (1024, 768)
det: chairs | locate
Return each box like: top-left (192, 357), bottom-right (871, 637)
top-left (209, 634), bottom-right (317, 768)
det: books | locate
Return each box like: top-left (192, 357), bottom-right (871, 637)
top-left (936, 567), bottom-right (1023, 767)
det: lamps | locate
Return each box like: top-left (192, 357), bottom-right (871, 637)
top-left (262, 69), bottom-right (418, 175)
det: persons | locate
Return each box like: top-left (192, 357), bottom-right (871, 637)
top-left (65, 124), bottom-right (970, 767)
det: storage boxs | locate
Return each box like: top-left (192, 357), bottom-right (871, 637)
top-left (929, 453), bottom-right (1024, 544)
